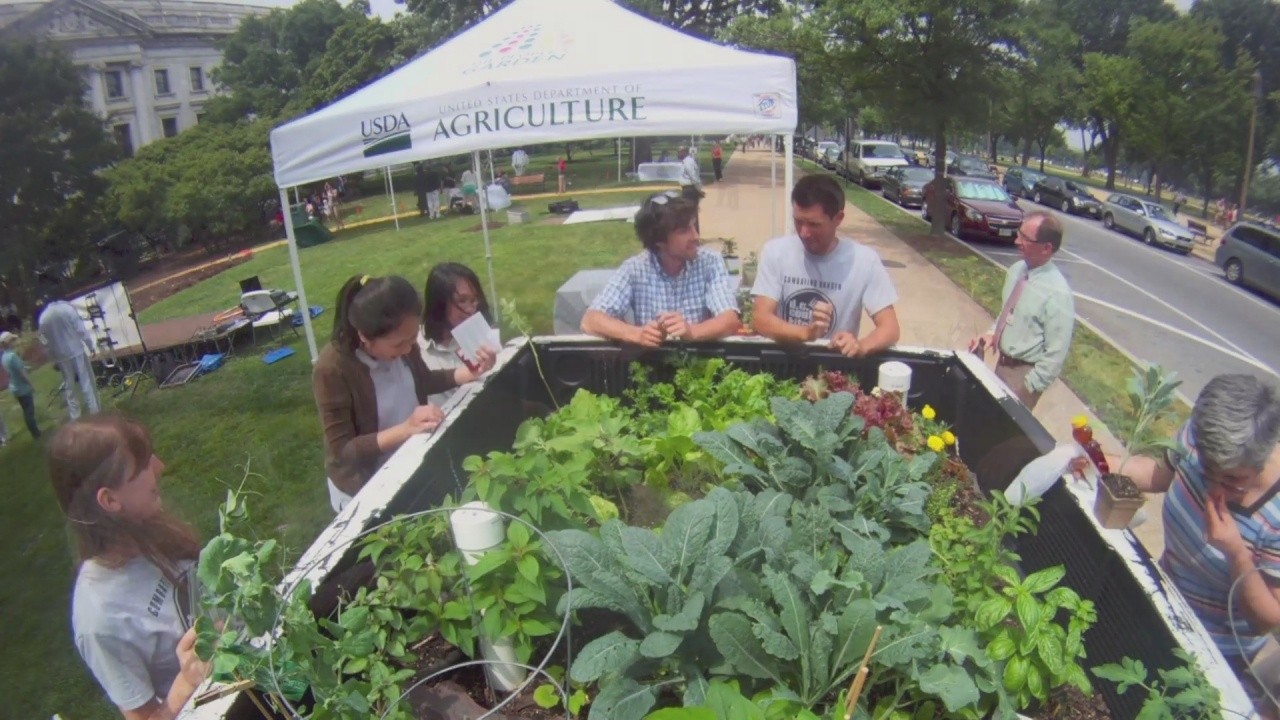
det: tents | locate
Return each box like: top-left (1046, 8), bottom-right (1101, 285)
top-left (269, 0), bottom-right (800, 367)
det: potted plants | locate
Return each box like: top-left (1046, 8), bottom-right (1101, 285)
top-left (1094, 361), bottom-right (1189, 529)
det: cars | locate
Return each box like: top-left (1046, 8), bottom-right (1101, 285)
top-left (948, 154), bottom-right (997, 181)
top-left (880, 164), bottom-right (935, 207)
top-left (1032, 175), bottom-right (1103, 221)
top-left (1100, 192), bottom-right (1194, 255)
top-left (926, 147), bottom-right (959, 173)
top-left (769, 134), bottom-right (930, 179)
top-left (1001, 166), bottom-right (1048, 201)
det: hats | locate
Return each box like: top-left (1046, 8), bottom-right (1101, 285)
top-left (0, 331), bottom-right (19, 342)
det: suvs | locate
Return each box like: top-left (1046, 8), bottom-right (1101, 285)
top-left (842, 137), bottom-right (910, 190)
top-left (1211, 220), bottom-right (1280, 307)
top-left (920, 175), bottom-right (1026, 244)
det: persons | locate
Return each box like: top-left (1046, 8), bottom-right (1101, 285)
top-left (1173, 190), bottom-right (1239, 232)
top-left (971, 211), bottom-right (1075, 412)
top-left (0, 330), bottom-right (47, 439)
top-left (747, 174), bottom-right (903, 356)
top-left (740, 133), bottom-right (761, 154)
top-left (555, 157), bottom-right (567, 193)
top-left (45, 410), bottom-right (231, 718)
top-left (710, 141), bottom-right (723, 182)
top-left (32, 282), bottom-right (102, 421)
top-left (412, 157), bottom-right (513, 222)
top-left (675, 142), bottom-right (706, 236)
top-left (578, 187), bottom-right (745, 347)
top-left (304, 176), bottom-right (347, 233)
top-left (311, 273), bottom-right (498, 516)
top-left (1075, 373), bottom-right (1279, 683)
top-left (418, 258), bottom-right (500, 408)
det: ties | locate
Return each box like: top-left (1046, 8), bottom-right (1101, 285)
top-left (989, 273), bottom-right (1026, 355)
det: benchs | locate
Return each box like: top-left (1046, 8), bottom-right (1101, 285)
top-left (508, 173), bottom-right (545, 194)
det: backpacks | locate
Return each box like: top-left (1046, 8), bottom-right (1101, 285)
top-left (538, 196), bottom-right (579, 214)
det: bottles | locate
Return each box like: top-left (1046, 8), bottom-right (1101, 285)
top-left (1070, 415), bottom-right (1110, 482)
top-left (973, 337), bottom-right (986, 361)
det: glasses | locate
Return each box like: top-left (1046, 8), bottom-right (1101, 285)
top-left (1017, 229), bottom-right (1039, 243)
top-left (648, 190), bottom-right (680, 208)
top-left (1202, 472), bottom-right (1248, 497)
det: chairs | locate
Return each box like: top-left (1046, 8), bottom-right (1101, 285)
top-left (240, 289), bottom-right (301, 350)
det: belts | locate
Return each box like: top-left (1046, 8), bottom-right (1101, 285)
top-left (999, 354), bottom-right (1034, 367)
